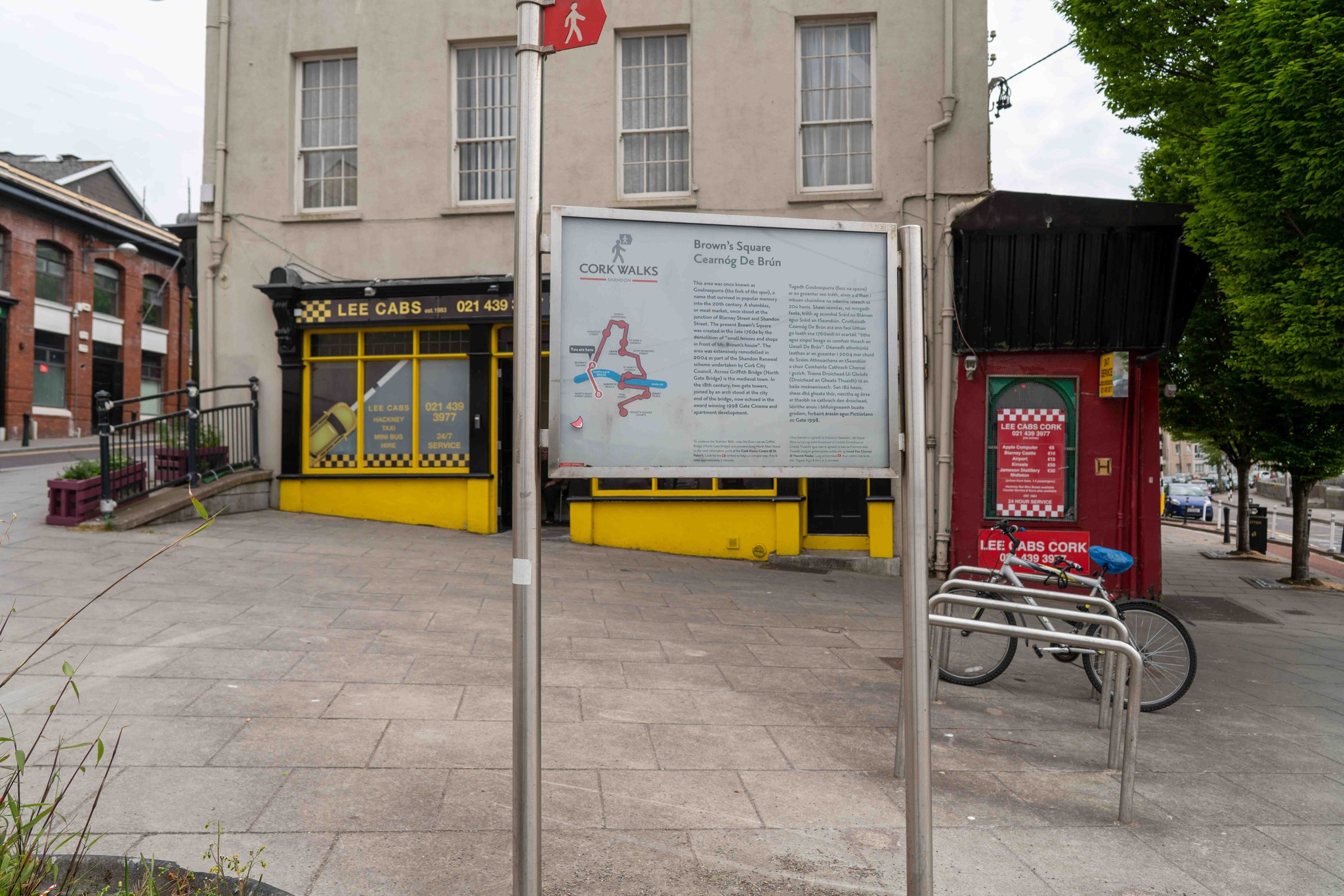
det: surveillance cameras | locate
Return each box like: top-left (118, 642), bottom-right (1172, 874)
top-left (1164, 384), bottom-right (1176, 397)
top-left (965, 356), bottom-right (977, 370)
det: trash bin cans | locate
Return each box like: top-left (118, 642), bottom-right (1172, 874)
top-left (1249, 506), bottom-right (1268, 555)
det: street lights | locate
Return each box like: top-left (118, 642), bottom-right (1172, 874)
top-left (73, 244), bottom-right (142, 439)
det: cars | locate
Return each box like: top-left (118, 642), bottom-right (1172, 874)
top-left (1161, 468), bottom-right (1283, 501)
top-left (1163, 482), bottom-right (1214, 521)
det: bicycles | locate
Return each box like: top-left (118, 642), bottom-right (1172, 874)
top-left (928, 513), bottom-right (1196, 712)
